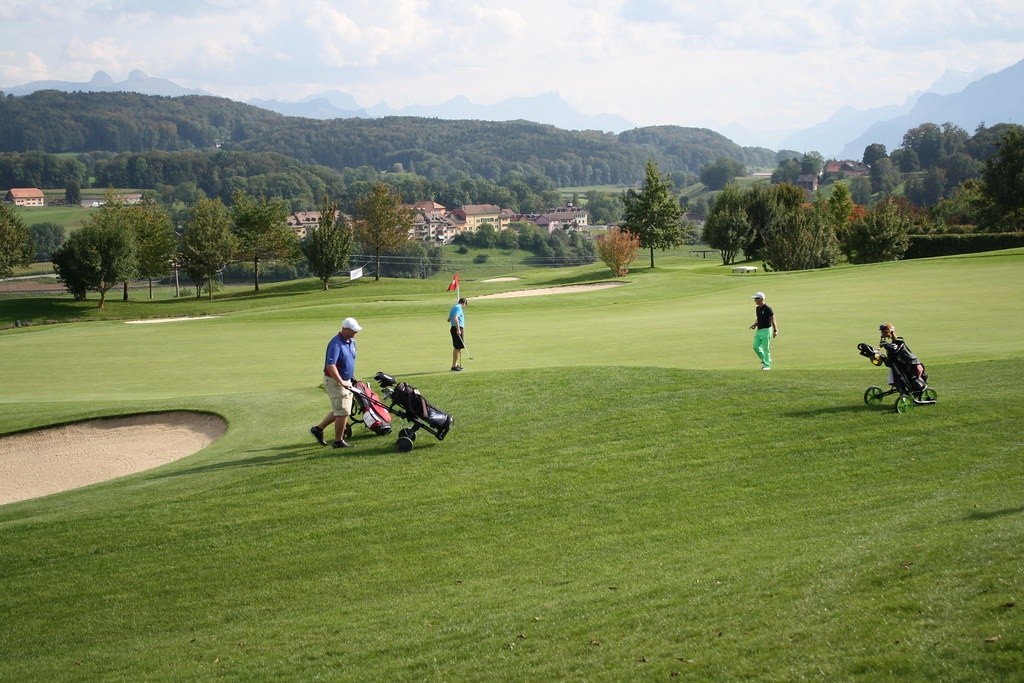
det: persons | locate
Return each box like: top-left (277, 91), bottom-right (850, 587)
top-left (749, 292), bottom-right (778, 370)
top-left (308, 317), bottom-right (363, 448)
top-left (447, 298), bottom-right (467, 372)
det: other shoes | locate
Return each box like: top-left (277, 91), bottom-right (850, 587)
top-left (763, 367), bottom-right (770, 370)
top-left (332, 440), bottom-right (350, 448)
top-left (451, 365), bottom-right (463, 371)
top-left (311, 426), bottom-right (327, 446)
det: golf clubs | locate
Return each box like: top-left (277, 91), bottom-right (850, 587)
top-left (459, 334), bottom-right (474, 359)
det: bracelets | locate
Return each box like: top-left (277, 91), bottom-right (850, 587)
top-left (774, 330), bottom-right (777, 332)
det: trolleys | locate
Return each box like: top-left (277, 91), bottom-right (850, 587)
top-left (857, 323), bottom-right (938, 414)
top-left (343, 372), bottom-right (454, 453)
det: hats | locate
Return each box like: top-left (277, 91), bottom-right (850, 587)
top-left (342, 318), bottom-right (363, 332)
top-left (752, 292), bottom-right (765, 298)
top-left (460, 298), bottom-right (467, 306)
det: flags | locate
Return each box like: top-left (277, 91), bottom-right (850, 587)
top-left (447, 273), bottom-right (459, 291)
